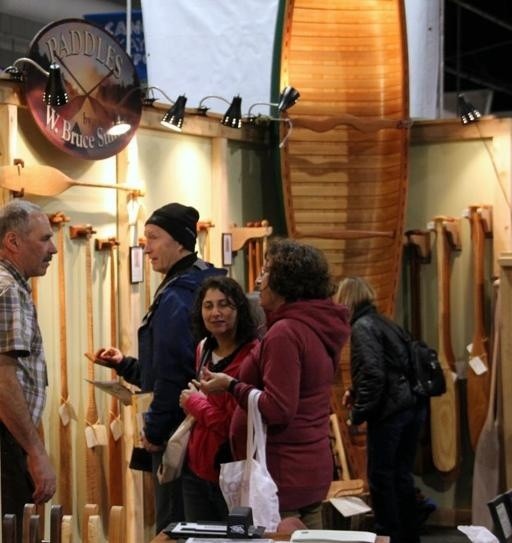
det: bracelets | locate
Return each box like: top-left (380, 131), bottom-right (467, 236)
top-left (228, 377), bottom-right (240, 394)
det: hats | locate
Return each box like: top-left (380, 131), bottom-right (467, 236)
top-left (143, 202), bottom-right (199, 252)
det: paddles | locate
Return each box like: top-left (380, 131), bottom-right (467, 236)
top-left (468, 205), bottom-right (490, 445)
top-left (430, 214), bottom-right (458, 471)
top-left (109, 238), bottom-right (123, 505)
top-left (85, 224), bottom-right (102, 516)
top-left (56, 212), bottom-right (72, 515)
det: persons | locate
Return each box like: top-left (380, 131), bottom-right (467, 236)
top-left (190, 236), bottom-right (352, 528)
top-left (85, 201), bottom-right (228, 536)
top-left (332, 274), bottom-right (421, 542)
top-left (0, 198), bottom-right (59, 542)
top-left (179, 274), bottom-right (264, 521)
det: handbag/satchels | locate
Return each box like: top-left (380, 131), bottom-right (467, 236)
top-left (219, 388), bottom-right (281, 533)
top-left (155, 337), bottom-right (207, 487)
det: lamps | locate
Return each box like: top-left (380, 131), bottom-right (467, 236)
top-left (246, 86), bottom-right (301, 118)
top-left (454, 90), bottom-right (481, 127)
top-left (144, 85), bottom-right (188, 133)
top-left (195, 93), bottom-right (244, 128)
top-left (3, 56), bottom-right (70, 106)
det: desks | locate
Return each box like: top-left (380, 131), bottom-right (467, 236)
top-left (149, 523), bottom-right (392, 542)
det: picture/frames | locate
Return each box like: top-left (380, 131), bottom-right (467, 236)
top-left (221, 232), bottom-right (233, 265)
top-left (129, 244), bottom-right (145, 284)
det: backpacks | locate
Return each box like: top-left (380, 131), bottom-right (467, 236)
top-left (367, 311), bottom-right (447, 397)
